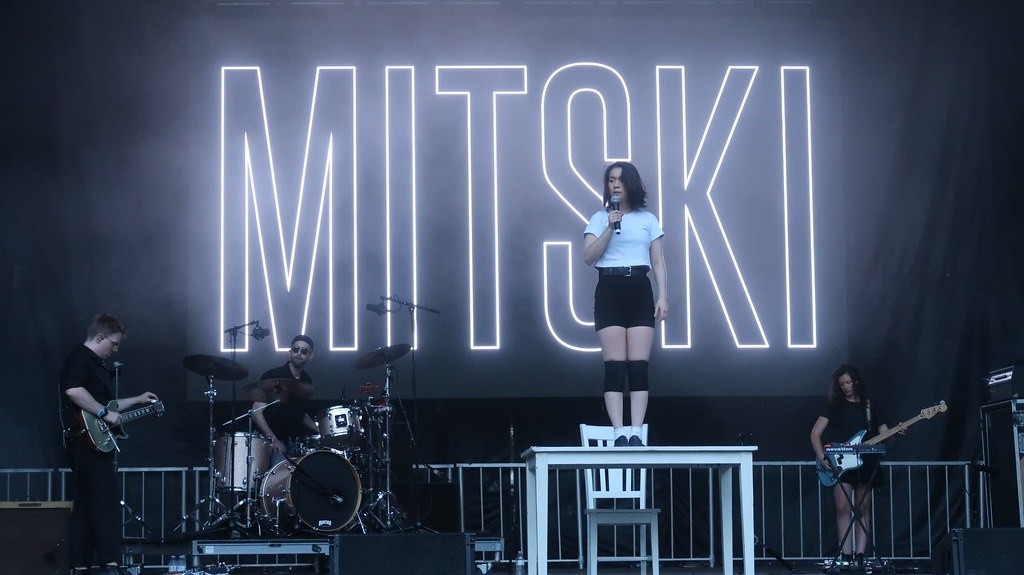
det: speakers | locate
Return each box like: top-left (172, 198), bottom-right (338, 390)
top-left (327, 534), bottom-right (477, 575)
top-left (0, 501), bottom-right (76, 575)
top-left (980, 399), bottom-right (1024, 528)
top-left (930, 527), bottom-right (1024, 575)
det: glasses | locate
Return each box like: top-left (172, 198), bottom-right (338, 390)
top-left (104, 336), bottom-right (119, 348)
top-left (292, 347), bottom-right (311, 354)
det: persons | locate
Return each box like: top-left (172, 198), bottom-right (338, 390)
top-left (250, 334), bottom-right (320, 467)
top-left (810, 363), bottom-right (908, 574)
top-left (60, 311), bottom-right (160, 575)
top-left (582, 160), bottom-right (670, 448)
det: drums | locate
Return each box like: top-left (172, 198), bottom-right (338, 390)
top-left (296, 434), bottom-right (322, 456)
top-left (214, 430), bottom-right (270, 493)
top-left (259, 449), bottom-right (363, 534)
top-left (315, 404), bottom-right (362, 448)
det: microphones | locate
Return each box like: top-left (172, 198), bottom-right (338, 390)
top-left (251, 328), bottom-right (270, 337)
top-left (367, 303), bottom-right (388, 312)
top-left (331, 494), bottom-right (344, 504)
top-left (610, 195), bottom-right (622, 234)
top-left (47, 545), bottom-right (60, 560)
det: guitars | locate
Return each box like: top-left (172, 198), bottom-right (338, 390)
top-left (815, 400), bottom-right (948, 488)
top-left (76, 399), bottom-right (165, 454)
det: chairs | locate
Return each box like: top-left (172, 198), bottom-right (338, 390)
top-left (581, 423), bottom-right (659, 575)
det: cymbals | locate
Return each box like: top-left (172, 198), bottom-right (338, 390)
top-left (243, 377), bottom-right (316, 402)
top-left (352, 344), bottom-right (411, 370)
top-left (182, 354), bottom-right (249, 382)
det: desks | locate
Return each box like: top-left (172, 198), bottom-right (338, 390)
top-left (521, 446), bottom-right (759, 574)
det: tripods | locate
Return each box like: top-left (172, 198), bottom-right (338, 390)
top-left (165, 320), bottom-right (281, 536)
top-left (349, 350), bottom-right (441, 535)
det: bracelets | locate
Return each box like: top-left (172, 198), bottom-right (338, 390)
top-left (97, 405), bottom-right (108, 418)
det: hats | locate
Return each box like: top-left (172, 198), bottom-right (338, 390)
top-left (292, 335), bottom-right (313, 346)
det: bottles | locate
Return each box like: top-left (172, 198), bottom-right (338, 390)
top-left (516, 551), bottom-right (525, 575)
top-left (168, 556), bottom-right (186, 575)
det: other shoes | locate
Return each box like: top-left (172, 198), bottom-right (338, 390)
top-left (72, 565), bottom-right (131, 575)
top-left (614, 435), bottom-right (645, 446)
